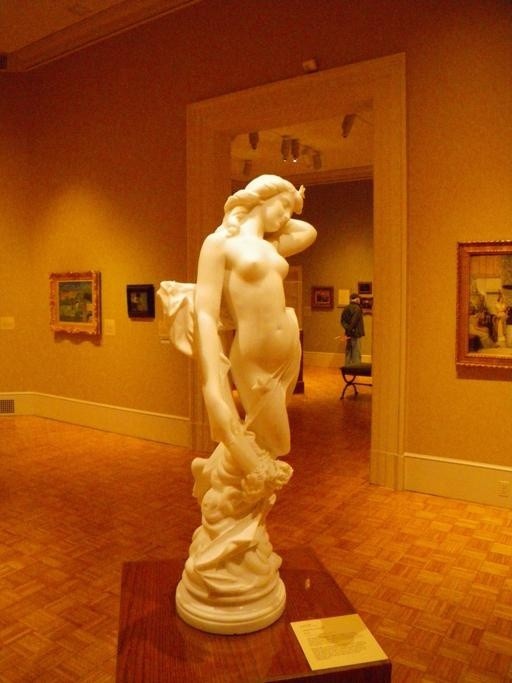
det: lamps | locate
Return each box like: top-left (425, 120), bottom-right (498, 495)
top-left (247, 131), bottom-right (323, 171)
top-left (341, 113), bottom-right (355, 138)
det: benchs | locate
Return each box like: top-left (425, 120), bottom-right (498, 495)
top-left (340, 363), bottom-right (371, 399)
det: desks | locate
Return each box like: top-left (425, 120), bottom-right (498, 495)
top-left (114, 543), bottom-right (393, 681)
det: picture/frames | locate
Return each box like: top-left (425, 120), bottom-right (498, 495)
top-left (454, 239), bottom-right (512, 369)
top-left (49, 269), bottom-right (102, 337)
top-left (126, 283), bottom-right (156, 318)
top-left (358, 281), bottom-right (374, 315)
top-left (311, 285), bottom-right (334, 308)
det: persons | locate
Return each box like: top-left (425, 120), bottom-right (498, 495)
top-left (338, 292), bottom-right (366, 367)
top-left (467, 274), bottom-right (507, 357)
top-left (188, 173), bottom-right (322, 521)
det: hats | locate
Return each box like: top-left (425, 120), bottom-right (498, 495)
top-left (349, 293), bottom-right (360, 299)
